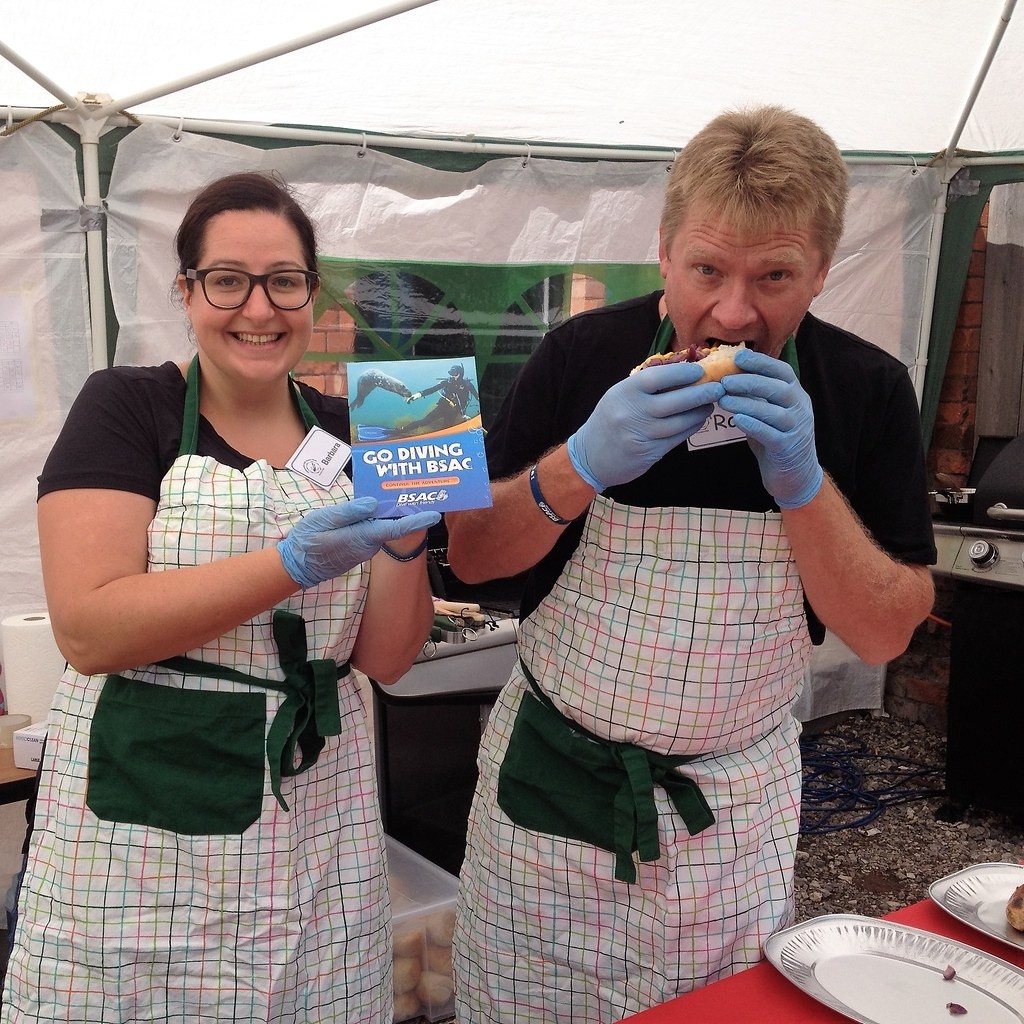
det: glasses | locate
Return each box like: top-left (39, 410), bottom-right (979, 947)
top-left (186, 267), bottom-right (317, 311)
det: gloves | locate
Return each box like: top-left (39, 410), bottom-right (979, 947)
top-left (567, 362), bottom-right (728, 494)
top-left (278, 496), bottom-right (441, 592)
top-left (718, 352), bottom-right (824, 510)
top-left (406, 392), bottom-right (422, 404)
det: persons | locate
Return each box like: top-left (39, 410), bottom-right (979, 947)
top-left (446, 103), bottom-right (938, 1024)
top-left (383, 362), bottom-right (479, 437)
top-left (0, 174), bottom-right (436, 1024)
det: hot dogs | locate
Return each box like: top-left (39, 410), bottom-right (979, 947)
top-left (628, 339), bottom-right (753, 393)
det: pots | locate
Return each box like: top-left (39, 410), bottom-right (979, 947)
top-left (928, 488), bottom-right (976, 514)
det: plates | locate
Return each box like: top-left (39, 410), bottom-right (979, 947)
top-left (928, 863), bottom-right (1024, 950)
top-left (763, 914), bottom-right (1024, 1024)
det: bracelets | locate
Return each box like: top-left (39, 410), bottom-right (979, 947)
top-left (380, 527), bottom-right (428, 562)
top-left (530, 460), bottom-right (573, 525)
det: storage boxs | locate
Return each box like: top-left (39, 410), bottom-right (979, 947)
top-left (13, 721), bottom-right (50, 771)
top-left (379, 832), bottom-right (459, 1023)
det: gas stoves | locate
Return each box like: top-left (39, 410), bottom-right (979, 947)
top-left (928, 511), bottom-right (1024, 586)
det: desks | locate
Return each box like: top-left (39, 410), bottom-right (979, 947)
top-left (612, 897), bottom-right (1024, 1024)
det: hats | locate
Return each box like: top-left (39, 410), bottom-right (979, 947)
top-left (448, 365), bottom-right (461, 376)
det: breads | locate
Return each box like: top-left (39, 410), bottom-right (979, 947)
top-left (1006, 883), bottom-right (1024, 932)
top-left (388, 908), bottom-right (456, 1022)
